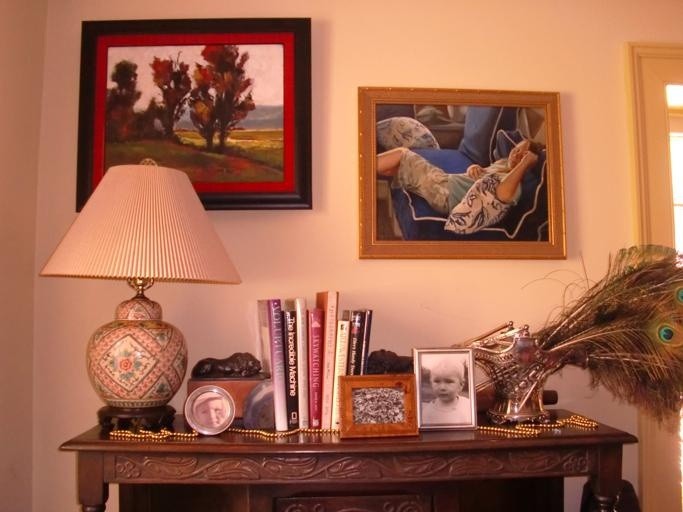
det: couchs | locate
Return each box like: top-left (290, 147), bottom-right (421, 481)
top-left (387, 104), bottom-right (549, 241)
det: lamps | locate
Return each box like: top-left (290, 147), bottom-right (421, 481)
top-left (40, 163), bottom-right (241, 435)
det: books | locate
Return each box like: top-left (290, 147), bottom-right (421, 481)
top-left (257, 291), bottom-right (373, 434)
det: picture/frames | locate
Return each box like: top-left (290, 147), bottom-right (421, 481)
top-left (337, 375), bottom-right (420, 438)
top-left (183, 384), bottom-right (235, 435)
top-left (356, 86), bottom-right (566, 260)
top-left (76, 17), bottom-right (313, 211)
top-left (411, 347), bottom-right (478, 431)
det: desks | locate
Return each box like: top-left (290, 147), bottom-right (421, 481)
top-left (58, 409), bottom-right (638, 512)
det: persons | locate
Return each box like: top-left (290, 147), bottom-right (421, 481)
top-left (421, 358), bottom-right (471, 424)
top-left (193, 392), bottom-right (227, 426)
top-left (376, 138), bottom-right (546, 235)
